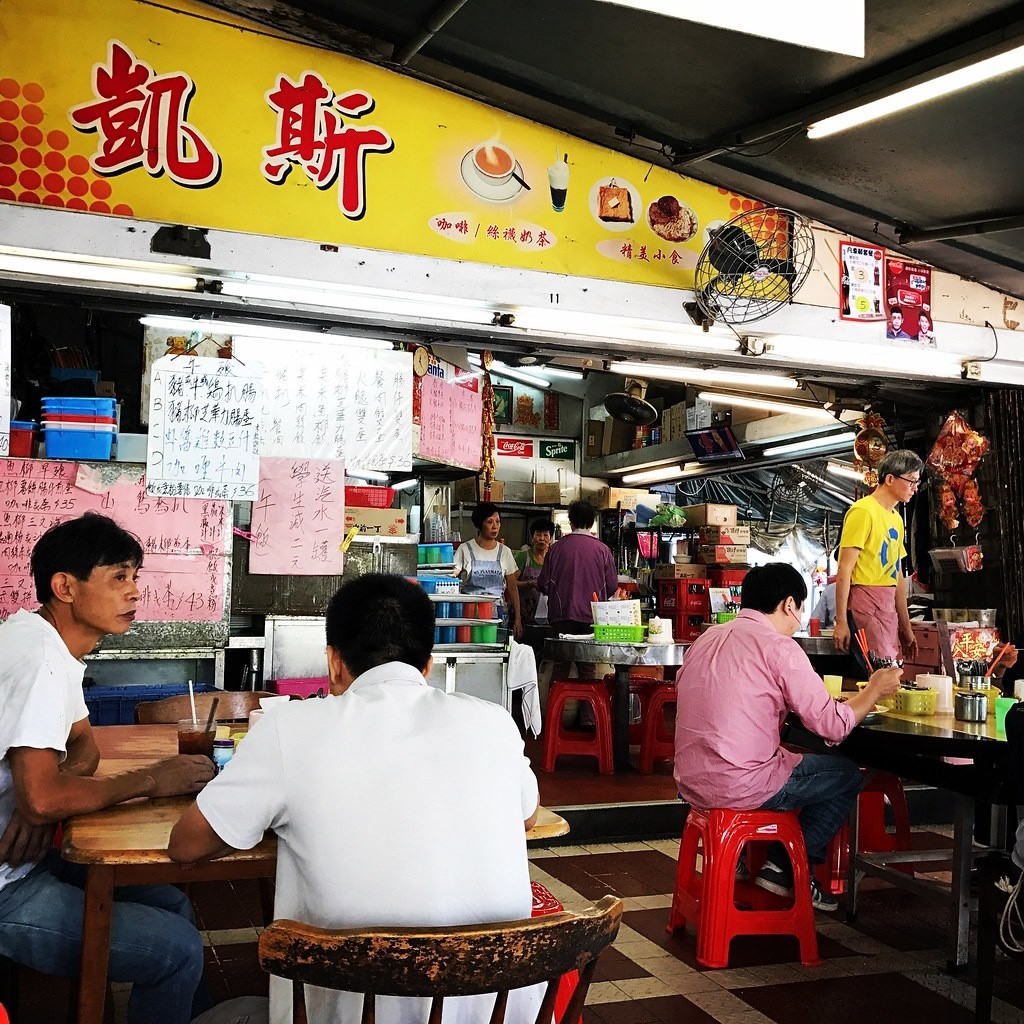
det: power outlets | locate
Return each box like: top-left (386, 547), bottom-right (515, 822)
top-left (741, 336), bottom-right (764, 356)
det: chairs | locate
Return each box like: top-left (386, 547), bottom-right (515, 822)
top-left (257, 895), bottom-right (622, 1024)
top-left (135, 689), bottom-right (287, 724)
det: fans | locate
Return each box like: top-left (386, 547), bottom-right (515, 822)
top-left (600, 380), bottom-right (658, 428)
top-left (681, 204), bottom-right (816, 326)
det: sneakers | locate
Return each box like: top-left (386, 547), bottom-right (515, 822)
top-left (735, 860), bottom-right (750, 881)
top-left (756, 862), bottom-right (838, 910)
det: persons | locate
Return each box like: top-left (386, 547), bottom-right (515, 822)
top-left (806, 547), bottom-right (839, 633)
top-left (673, 563), bottom-right (904, 911)
top-left (833, 450), bottom-right (924, 662)
top-left (887, 307), bottom-right (910, 338)
top-left (507, 519), bottom-right (555, 644)
top-left (538, 500), bottom-right (619, 724)
top-left (912, 311), bottom-right (933, 343)
top-left (453, 502), bottom-right (522, 639)
top-left (1, 512), bottom-right (216, 1023)
top-left (166, 574), bottom-right (556, 1023)
top-left (1005, 702), bottom-right (1024, 872)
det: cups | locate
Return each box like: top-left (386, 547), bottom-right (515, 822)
top-left (995, 698), bottom-right (1019, 732)
top-left (418, 546), bottom-right (453, 564)
top-left (823, 674), bottom-right (842, 699)
top-left (435, 603), bottom-right (495, 619)
top-left (810, 619), bottom-right (820, 637)
top-left (434, 626), bottom-right (497, 643)
top-left (177, 718), bottom-right (218, 765)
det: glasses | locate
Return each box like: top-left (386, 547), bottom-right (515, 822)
top-left (896, 475), bottom-right (922, 487)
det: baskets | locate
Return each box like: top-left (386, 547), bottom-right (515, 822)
top-left (404, 574), bottom-right (463, 594)
top-left (717, 612), bottom-right (737, 624)
top-left (857, 680), bottom-right (940, 716)
top-left (591, 624), bottom-right (648, 643)
top-left (953, 683), bottom-right (1002, 713)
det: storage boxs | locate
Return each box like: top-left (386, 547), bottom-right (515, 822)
top-left (85, 683), bottom-right (226, 725)
top-left (590, 625), bottom-right (649, 642)
top-left (42, 394), bottom-right (148, 464)
top-left (479, 479), bottom-right (504, 501)
top-left (9, 421), bottom-right (39, 457)
top-left (534, 482), bottom-right (561, 505)
top-left (585, 401), bottom-right (781, 643)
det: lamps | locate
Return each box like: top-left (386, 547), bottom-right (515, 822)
top-left (0, 244), bottom-right (1024, 483)
top-left (801, 34), bottom-right (1024, 142)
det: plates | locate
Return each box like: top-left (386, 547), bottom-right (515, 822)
top-left (866, 705), bottom-right (889, 717)
top-left (459, 148), bottom-right (524, 201)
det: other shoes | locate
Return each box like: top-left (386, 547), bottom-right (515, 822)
top-left (581, 718), bottom-right (595, 726)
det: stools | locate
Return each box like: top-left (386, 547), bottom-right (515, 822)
top-left (532, 673), bottom-right (914, 1024)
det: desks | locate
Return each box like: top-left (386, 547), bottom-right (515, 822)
top-left (68, 722), bottom-right (569, 1023)
top-left (541, 635), bottom-right (689, 779)
top-left (797, 697), bottom-right (1010, 969)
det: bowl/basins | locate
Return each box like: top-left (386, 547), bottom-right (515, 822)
top-left (472, 142), bottom-right (516, 186)
top-left (672, 556), bottom-right (692, 564)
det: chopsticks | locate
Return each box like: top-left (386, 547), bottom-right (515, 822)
top-left (854, 628), bottom-right (876, 674)
top-left (984, 642), bottom-right (1010, 676)
